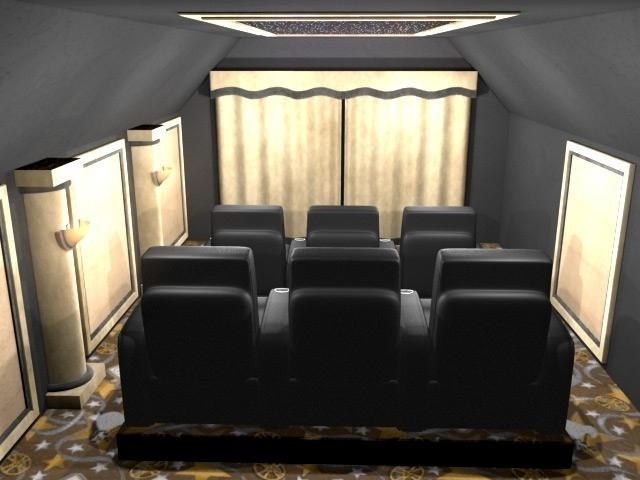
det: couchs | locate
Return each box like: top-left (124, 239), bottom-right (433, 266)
top-left (287, 205), bottom-right (398, 286)
top-left (205, 206), bottom-right (286, 288)
top-left (399, 243), bottom-right (573, 425)
top-left (263, 245), bottom-right (404, 427)
top-left (120, 242), bottom-right (262, 426)
top-left (398, 206), bottom-right (481, 300)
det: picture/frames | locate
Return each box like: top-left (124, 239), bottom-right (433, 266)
top-left (549, 140), bottom-right (636, 363)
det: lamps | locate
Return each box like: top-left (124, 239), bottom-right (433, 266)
top-left (55, 218), bottom-right (88, 251)
top-left (151, 165), bottom-right (171, 187)
